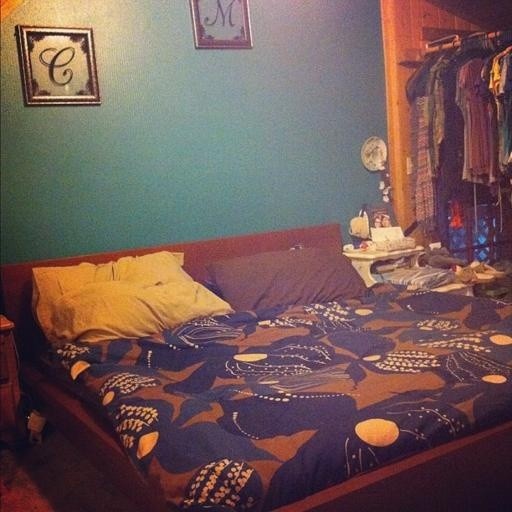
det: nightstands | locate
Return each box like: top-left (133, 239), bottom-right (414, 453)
top-left (340, 246), bottom-right (474, 296)
top-left (0, 314), bottom-right (31, 448)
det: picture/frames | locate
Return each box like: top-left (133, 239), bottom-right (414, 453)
top-left (14, 23), bottom-right (102, 107)
top-left (188, 0), bottom-right (254, 51)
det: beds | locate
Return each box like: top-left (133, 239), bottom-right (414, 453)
top-left (1, 224), bottom-right (510, 512)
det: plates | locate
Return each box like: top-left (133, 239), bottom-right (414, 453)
top-left (360, 136), bottom-right (387, 173)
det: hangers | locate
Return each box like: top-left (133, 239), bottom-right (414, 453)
top-left (406, 27), bottom-right (511, 94)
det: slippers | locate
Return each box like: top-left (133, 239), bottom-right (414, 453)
top-left (463, 261), bottom-right (504, 279)
top-left (454, 267), bottom-right (496, 284)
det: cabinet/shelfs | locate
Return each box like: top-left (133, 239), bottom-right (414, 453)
top-left (378, 1), bottom-right (511, 301)
